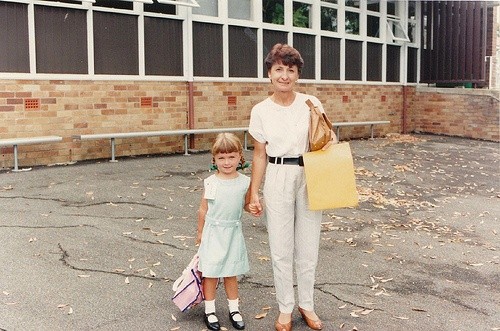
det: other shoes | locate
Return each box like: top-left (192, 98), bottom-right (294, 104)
top-left (228, 311), bottom-right (245, 330)
top-left (203, 312), bottom-right (220, 331)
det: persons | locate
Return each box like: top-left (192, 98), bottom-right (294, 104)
top-left (247, 43), bottom-right (337, 331)
top-left (194, 133), bottom-right (258, 330)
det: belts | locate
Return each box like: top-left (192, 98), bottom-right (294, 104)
top-left (268, 155), bottom-right (304, 166)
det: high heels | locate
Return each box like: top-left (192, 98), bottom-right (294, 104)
top-left (297, 305), bottom-right (324, 330)
top-left (274, 312), bottom-right (292, 331)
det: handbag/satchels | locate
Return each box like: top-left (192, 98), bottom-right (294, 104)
top-left (305, 99), bottom-right (333, 152)
top-left (301, 141), bottom-right (359, 211)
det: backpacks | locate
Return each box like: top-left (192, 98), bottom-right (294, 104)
top-left (172, 243), bottom-right (221, 313)
